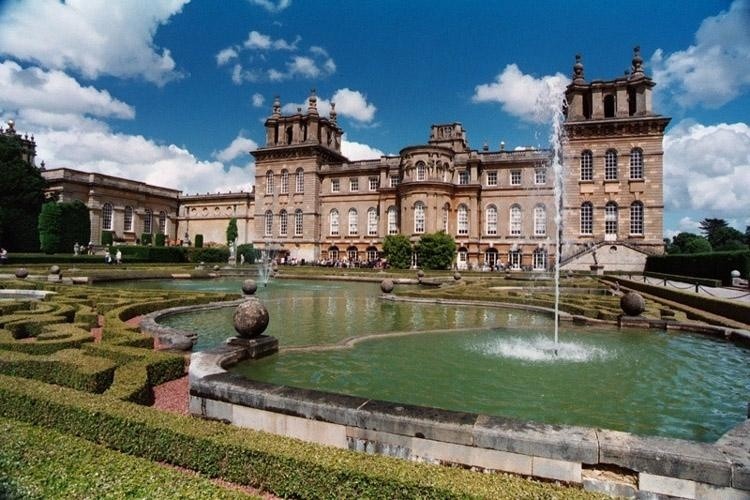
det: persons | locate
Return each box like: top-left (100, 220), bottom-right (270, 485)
top-left (73, 241), bottom-right (96, 256)
top-left (240, 254), bottom-right (387, 268)
top-left (0, 247), bottom-right (8, 265)
top-left (105, 249), bottom-right (122, 266)
top-left (453, 256), bottom-right (520, 270)
